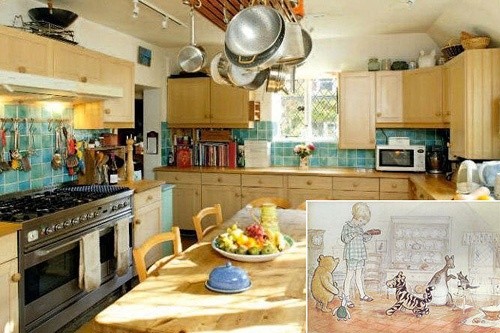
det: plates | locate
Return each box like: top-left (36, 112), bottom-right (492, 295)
top-left (205, 278), bottom-right (253, 293)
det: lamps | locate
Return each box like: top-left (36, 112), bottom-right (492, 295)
top-left (132, 0), bottom-right (140, 17)
top-left (160, 15), bottom-right (169, 27)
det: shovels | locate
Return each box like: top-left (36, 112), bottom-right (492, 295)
top-left (63, 127), bottom-right (79, 167)
top-left (20, 119), bottom-right (32, 172)
top-left (0, 128), bottom-right (10, 171)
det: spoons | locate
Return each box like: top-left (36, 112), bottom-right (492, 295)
top-left (10, 119), bottom-right (22, 159)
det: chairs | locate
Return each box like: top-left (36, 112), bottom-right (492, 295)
top-left (248, 195), bottom-right (289, 210)
top-left (296, 198), bottom-right (332, 209)
top-left (133, 226), bottom-right (183, 282)
top-left (192, 202), bottom-right (222, 241)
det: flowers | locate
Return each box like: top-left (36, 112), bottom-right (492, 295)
top-left (294, 142), bottom-right (314, 157)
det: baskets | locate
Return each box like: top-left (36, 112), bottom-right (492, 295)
top-left (461, 37), bottom-right (490, 49)
top-left (440, 43), bottom-right (464, 61)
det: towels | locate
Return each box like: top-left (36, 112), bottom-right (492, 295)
top-left (77, 230), bottom-right (102, 291)
top-left (113, 217), bottom-right (129, 277)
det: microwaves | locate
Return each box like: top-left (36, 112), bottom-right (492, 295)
top-left (375, 144), bottom-right (427, 173)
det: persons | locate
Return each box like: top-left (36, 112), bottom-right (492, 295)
top-left (340, 202), bottom-right (374, 308)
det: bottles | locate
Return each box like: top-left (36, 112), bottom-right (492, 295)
top-left (368, 57), bottom-right (380, 70)
top-left (107, 152), bottom-right (118, 184)
top-left (409, 61), bottom-right (416, 69)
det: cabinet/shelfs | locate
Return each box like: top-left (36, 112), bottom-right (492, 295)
top-left (1, 228), bottom-right (20, 333)
top-left (338, 71), bottom-right (376, 149)
top-left (201, 173), bottom-right (286, 230)
top-left (410, 181), bottom-right (435, 199)
top-left (402, 66), bottom-right (450, 123)
top-left (78, 144), bottom-right (126, 183)
top-left (0, 27), bottom-right (101, 83)
top-left (286, 175), bottom-right (379, 210)
top-left (157, 171), bottom-right (201, 230)
top-left (380, 178), bottom-right (410, 199)
top-left (132, 184), bottom-right (162, 277)
top-left (162, 182), bottom-right (175, 256)
top-left (166, 77), bottom-right (260, 129)
top-left (375, 71), bottom-right (402, 123)
top-left (450, 49), bottom-right (500, 159)
top-left (74, 51), bottom-right (135, 129)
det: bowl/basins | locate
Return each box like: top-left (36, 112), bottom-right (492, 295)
top-left (212, 228), bottom-right (295, 262)
top-left (480, 306), bottom-right (500, 320)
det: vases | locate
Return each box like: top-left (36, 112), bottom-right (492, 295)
top-left (299, 157), bottom-right (309, 168)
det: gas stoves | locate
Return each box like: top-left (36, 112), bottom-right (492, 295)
top-left (0, 185), bottom-right (129, 223)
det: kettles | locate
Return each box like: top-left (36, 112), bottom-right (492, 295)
top-left (457, 159), bottom-right (481, 194)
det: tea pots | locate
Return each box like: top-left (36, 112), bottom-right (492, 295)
top-left (250, 203), bottom-right (281, 232)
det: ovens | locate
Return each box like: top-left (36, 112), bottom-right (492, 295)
top-left (19, 197), bottom-right (132, 332)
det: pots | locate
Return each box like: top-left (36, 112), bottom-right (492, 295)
top-left (226, 62), bottom-right (256, 86)
top-left (267, 64), bottom-right (289, 94)
top-left (243, 68), bottom-right (268, 90)
top-left (177, 13), bottom-right (207, 72)
top-left (209, 51), bottom-right (231, 87)
top-left (224, 0), bottom-right (283, 62)
top-left (225, 12), bottom-right (286, 69)
top-left (28, 0), bottom-right (78, 28)
top-left (285, 29), bottom-right (313, 68)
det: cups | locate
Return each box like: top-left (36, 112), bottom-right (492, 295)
top-left (478, 160), bottom-right (500, 186)
top-left (381, 58), bottom-right (391, 69)
